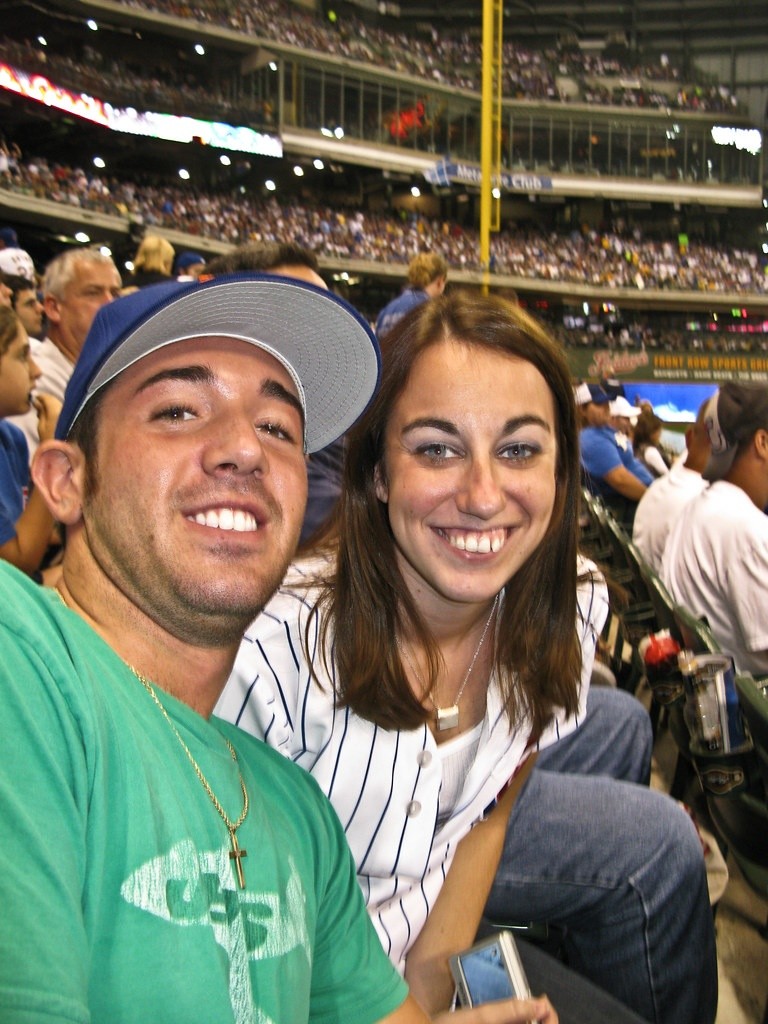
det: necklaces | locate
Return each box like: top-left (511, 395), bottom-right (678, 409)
top-left (394, 593), bottom-right (499, 734)
top-left (124, 657), bottom-right (250, 890)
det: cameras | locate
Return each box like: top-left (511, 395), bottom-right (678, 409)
top-left (449, 929), bottom-right (538, 1024)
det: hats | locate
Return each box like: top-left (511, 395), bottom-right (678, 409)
top-left (50, 267), bottom-right (382, 449)
top-left (0, 228), bottom-right (19, 244)
top-left (173, 252), bottom-right (206, 275)
top-left (574, 381), bottom-right (620, 406)
top-left (701, 378), bottom-right (768, 481)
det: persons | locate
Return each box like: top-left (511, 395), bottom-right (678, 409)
top-left (0, 295), bottom-right (558, 1023)
top-left (0, 0), bottom-right (768, 682)
top-left (206, 242), bottom-right (717, 1024)
top-left (209, 290), bottom-right (649, 1024)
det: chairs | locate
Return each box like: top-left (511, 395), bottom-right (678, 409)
top-left (577, 488), bottom-right (768, 944)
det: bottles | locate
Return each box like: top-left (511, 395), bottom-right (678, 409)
top-left (678, 651), bottom-right (735, 796)
